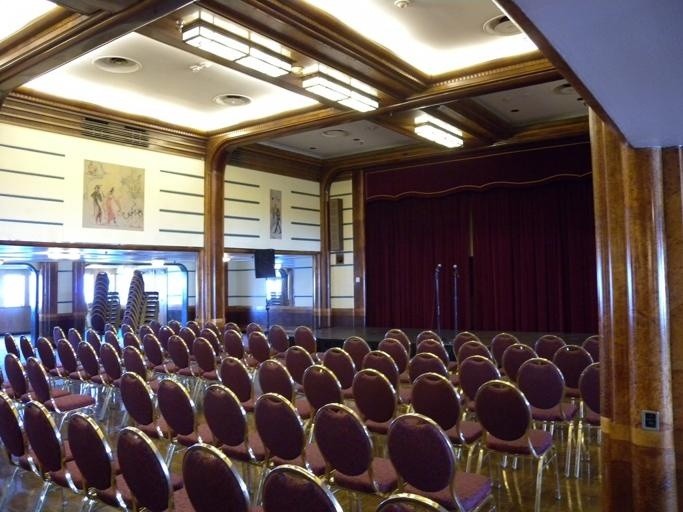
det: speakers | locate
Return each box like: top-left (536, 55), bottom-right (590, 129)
top-left (255, 249), bottom-right (275, 278)
top-left (329, 198), bottom-right (343, 251)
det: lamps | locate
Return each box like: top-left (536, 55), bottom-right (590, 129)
top-left (179, 8), bottom-right (297, 78)
top-left (300, 61), bottom-right (384, 113)
top-left (413, 113), bottom-right (464, 149)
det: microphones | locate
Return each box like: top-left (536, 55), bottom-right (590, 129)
top-left (434, 263), bottom-right (442, 274)
top-left (453, 264), bottom-right (460, 277)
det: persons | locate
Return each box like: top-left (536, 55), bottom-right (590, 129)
top-left (273, 208), bottom-right (281, 234)
top-left (91, 185), bottom-right (103, 224)
top-left (102, 187), bottom-right (121, 223)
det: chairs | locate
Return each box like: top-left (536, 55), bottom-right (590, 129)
top-left (0, 320), bottom-right (600, 512)
top-left (91, 265), bottom-right (162, 332)
